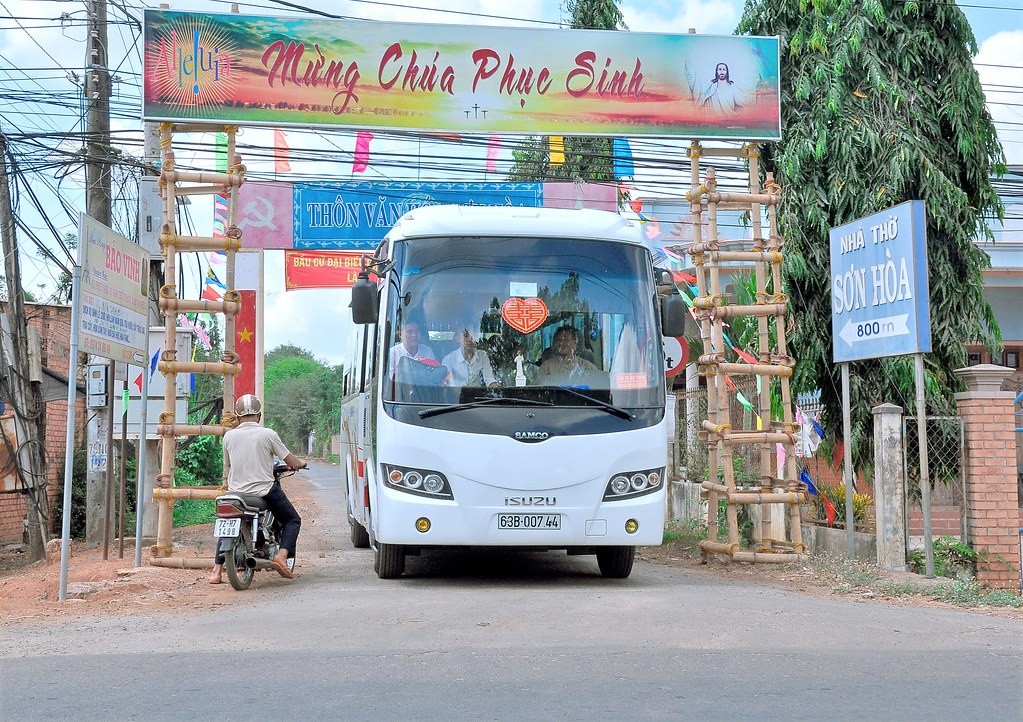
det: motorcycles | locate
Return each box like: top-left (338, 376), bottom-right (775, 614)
top-left (212, 460), bottom-right (309, 592)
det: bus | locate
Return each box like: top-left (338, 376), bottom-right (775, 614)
top-left (338, 203), bottom-right (686, 579)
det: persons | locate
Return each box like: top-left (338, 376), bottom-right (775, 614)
top-left (206, 395), bottom-right (307, 583)
top-left (512, 350), bottom-right (525, 376)
top-left (389, 320), bottom-right (443, 383)
top-left (442, 323), bottom-right (501, 389)
top-left (537, 326), bottom-right (596, 376)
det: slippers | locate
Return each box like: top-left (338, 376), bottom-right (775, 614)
top-left (271, 561), bottom-right (294, 579)
top-left (208, 576), bottom-right (221, 584)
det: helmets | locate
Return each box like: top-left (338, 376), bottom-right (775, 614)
top-left (234, 394), bottom-right (262, 417)
